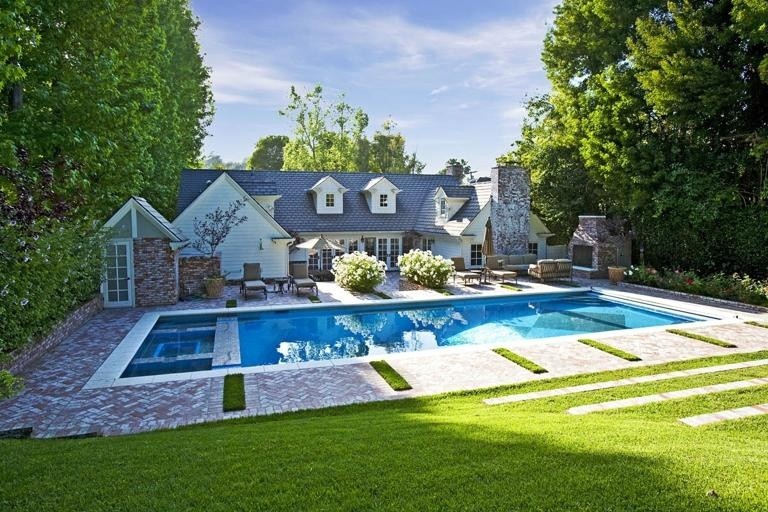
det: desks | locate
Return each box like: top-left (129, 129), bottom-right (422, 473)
top-left (470, 270), bottom-right (492, 284)
top-left (272, 279), bottom-right (288, 294)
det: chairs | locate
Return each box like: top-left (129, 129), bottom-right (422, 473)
top-left (484, 256), bottom-right (518, 287)
top-left (239, 262), bottom-right (268, 300)
top-left (451, 256), bottom-right (482, 286)
top-left (288, 263), bottom-right (318, 297)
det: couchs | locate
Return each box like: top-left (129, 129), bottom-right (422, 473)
top-left (527, 258), bottom-right (574, 284)
top-left (495, 254), bottom-right (537, 279)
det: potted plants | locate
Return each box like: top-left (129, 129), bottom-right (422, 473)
top-left (605, 252), bottom-right (627, 285)
top-left (192, 195), bottom-right (248, 297)
